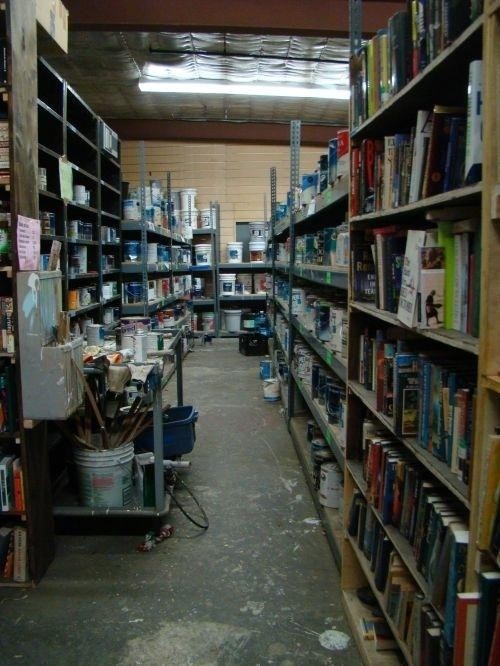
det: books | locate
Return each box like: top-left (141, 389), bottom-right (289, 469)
top-left (348, 61), bottom-right (483, 218)
top-left (348, 419), bottom-right (500, 666)
top-left (349, 0), bottom-right (484, 133)
top-left (358, 328), bottom-right (475, 485)
top-left (349, 206), bottom-right (481, 337)
top-left (2, 292), bottom-right (31, 584)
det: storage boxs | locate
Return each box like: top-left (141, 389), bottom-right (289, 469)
top-left (132, 404), bottom-right (199, 459)
top-left (0, 0), bottom-right (70, 56)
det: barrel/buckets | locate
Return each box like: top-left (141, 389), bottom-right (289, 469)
top-left (254, 310), bottom-right (270, 338)
top-left (291, 337), bottom-right (346, 437)
top-left (123, 177), bottom-right (198, 239)
top-left (253, 273), bottom-right (268, 294)
top-left (200, 208), bottom-right (216, 229)
top-left (263, 378), bottom-right (281, 402)
top-left (194, 243), bottom-right (212, 266)
top-left (237, 273), bottom-right (252, 295)
top-left (192, 277), bottom-right (206, 297)
top-left (248, 222), bottom-right (266, 242)
top-left (201, 312), bottom-right (216, 331)
top-left (123, 385), bottom-right (141, 407)
top-left (34, 163), bottom-right (120, 349)
top-left (123, 274), bottom-right (192, 306)
top-left (268, 267), bottom-right (348, 359)
top-left (192, 313), bottom-right (198, 331)
top-left (259, 360), bottom-right (274, 380)
top-left (241, 309), bottom-right (256, 331)
top-left (73, 433), bottom-right (134, 507)
top-left (122, 234), bottom-right (191, 265)
top-left (220, 308), bottom-right (226, 330)
top-left (290, 127), bottom-right (350, 206)
top-left (120, 300), bottom-right (189, 362)
top-left (226, 241), bottom-right (243, 263)
top-left (224, 308), bottom-right (242, 332)
top-left (304, 419), bottom-right (346, 509)
top-left (249, 241), bottom-right (266, 264)
top-left (276, 221), bottom-right (352, 267)
top-left (219, 273), bottom-right (236, 296)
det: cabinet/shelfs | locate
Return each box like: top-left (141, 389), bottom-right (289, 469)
top-left (0, 45), bottom-right (124, 589)
top-left (289, 116), bottom-right (348, 573)
top-left (122, 140), bottom-right (190, 361)
top-left (344, 0), bottom-right (500, 665)
top-left (269, 164), bottom-right (290, 416)
top-left (188, 196), bottom-right (215, 340)
top-left (215, 200), bottom-right (267, 339)
